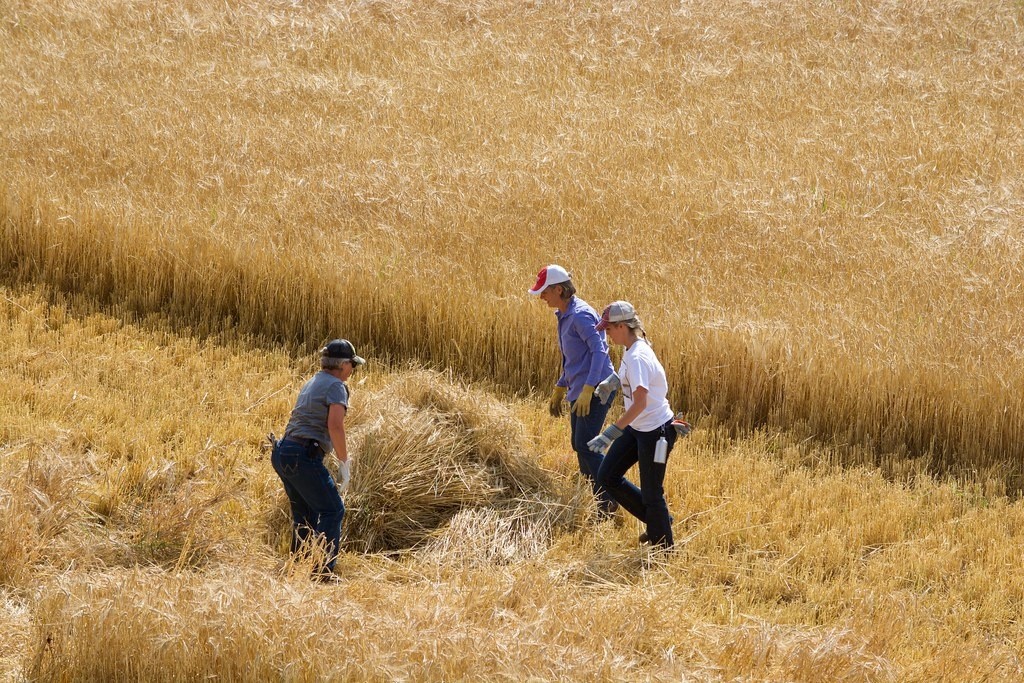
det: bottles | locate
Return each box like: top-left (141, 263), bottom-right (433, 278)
top-left (654, 431), bottom-right (668, 463)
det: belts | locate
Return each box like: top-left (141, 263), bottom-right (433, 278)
top-left (284, 435), bottom-right (324, 456)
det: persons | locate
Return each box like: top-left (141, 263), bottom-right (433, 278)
top-left (586, 301), bottom-right (676, 552)
top-left (527, 264), bottom-right (617, 520)
top-left (270, 338), bottom-right (366, 583)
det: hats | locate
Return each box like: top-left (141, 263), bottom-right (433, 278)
top-left (528, 264), bottom-right (573, 295)
top-left (321, 338), bottom-right (366, 364)
top-left (593, 301), bottom-right (636, 331)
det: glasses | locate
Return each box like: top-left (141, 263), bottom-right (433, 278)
top-left (349, 360), bottom-right (357, 367)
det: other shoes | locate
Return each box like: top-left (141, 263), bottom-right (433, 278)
top-left (638, 529), bottom-right (649, 543)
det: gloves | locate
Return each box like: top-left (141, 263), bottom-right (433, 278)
top-left (587, 424), bottom-right (624, 453)
top-left (571, 383), bottom-right (595, 418)
top-left (546, 385), bottom-right (568, 418)
top-left (594, 371), bottom-right (622, 407)
top-left (336, 458), bottom-right (351, 495)
